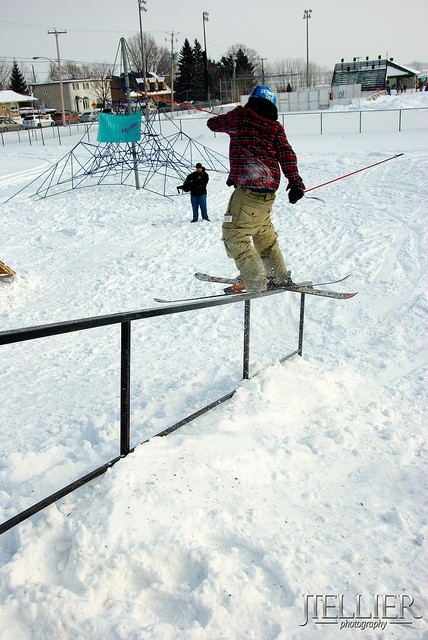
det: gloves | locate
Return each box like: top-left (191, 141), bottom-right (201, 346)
top-left (288, 187), bottom-right (303, 204)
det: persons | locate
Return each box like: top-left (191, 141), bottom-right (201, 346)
top-left (207, 84), bottom-right (306, 295)
top-left (384, 75), bottom-right (427, 94)
top-left (179, 164), bottom-right (211, 223)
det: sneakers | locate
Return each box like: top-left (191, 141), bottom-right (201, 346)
top-left (203, 218), bottom-right (210, 221)
top-left (223, 279), bottom-right (251, 294)
top-left (191, 218), bottom-right (197, 222)
top-left (267, 275), bottom-right (292, 287)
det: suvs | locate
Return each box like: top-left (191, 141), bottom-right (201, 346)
top-left (22, 114), bottom-right (56, 130)
top-left (51, 112), bottom-right (82, 126)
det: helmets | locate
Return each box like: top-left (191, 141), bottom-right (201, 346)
top-left (247, 85), bottom-right (276, 106)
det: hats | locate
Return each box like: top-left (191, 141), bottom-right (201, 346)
top-left (196, 163), bottom-right (202, 168)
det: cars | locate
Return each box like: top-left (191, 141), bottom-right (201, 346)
top-left (102, 107), bottom-right (126, 117)
top-left (78, 111), bottom-right (100, 122)
top-left (0, 118), bottom-right (25, 133)
top-left (142, 104), bottom-right (159, 114)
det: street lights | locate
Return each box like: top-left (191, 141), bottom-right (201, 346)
top-left (301, 7), bottom-right (312, 88)
top-left (31, 30), bottom-right (69, 125)
top-left (137, 0), bottom-right (149, 116)
top-left (202, 10), bottom-right (213, 109)
